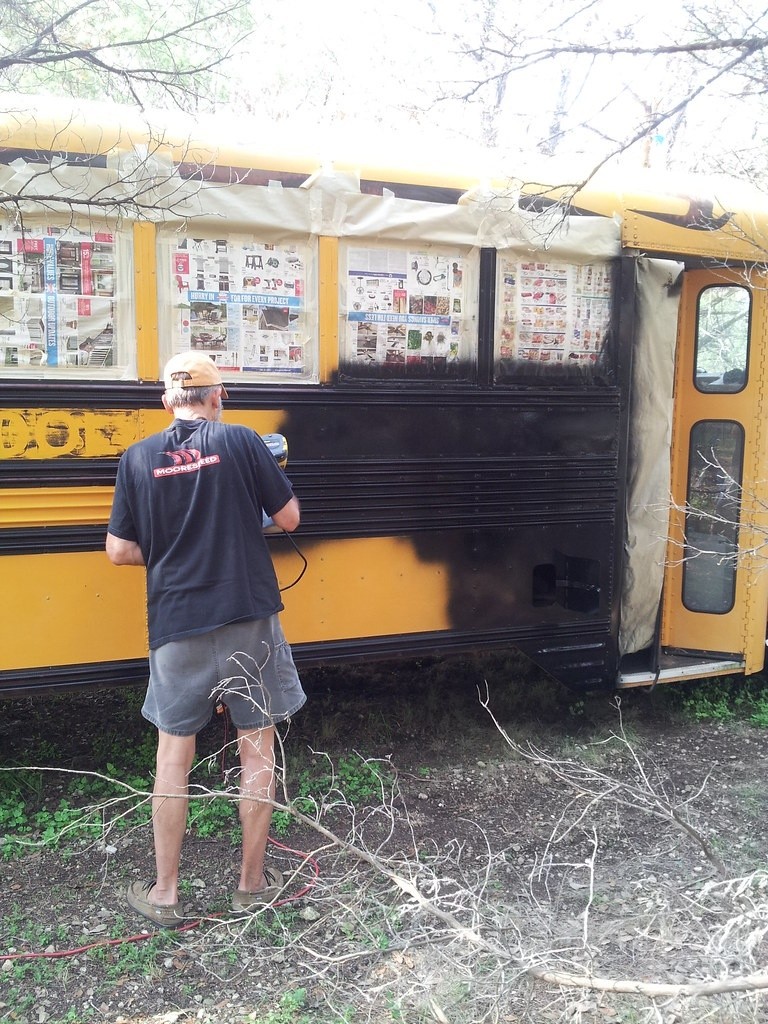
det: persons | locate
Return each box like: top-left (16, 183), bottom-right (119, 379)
top-left (105, 351), bottom-right (309, 927)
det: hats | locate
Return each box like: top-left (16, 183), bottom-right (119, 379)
top-left (164, 351), bottom-right (229, 399)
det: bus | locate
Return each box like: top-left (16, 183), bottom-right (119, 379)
top-left (0, 91), bottom-right (768, 692)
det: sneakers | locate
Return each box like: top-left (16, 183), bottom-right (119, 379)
top-left (126, 880), bottom-right (184, 929)
top-left (232, 868), bottom-right (284, 917)
